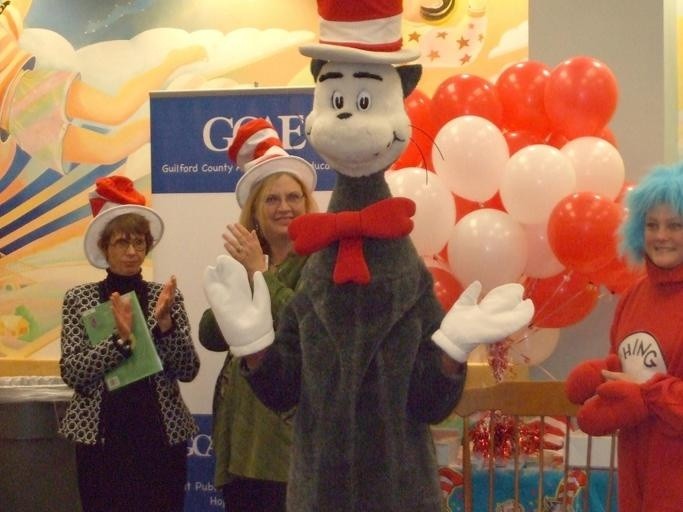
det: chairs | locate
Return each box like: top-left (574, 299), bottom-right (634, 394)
top-left (453, 379), bottom-right (619, 512)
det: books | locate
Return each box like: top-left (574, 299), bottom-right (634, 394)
top-left (78, 290), bottom-right (163, 391)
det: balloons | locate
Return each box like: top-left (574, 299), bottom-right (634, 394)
top-left (522, 269), bottom-right (600, 329)
top-left (424, 266), bottom-right (465, 314)
top-left (544, 55), bottom-right (618, 139)
top-left (600, 257), bottom-right (645, 295)
top-left (560, 137), bottom-right (625, 201)
top-left (616, 181), bottom-right (635, 202)
top-left (432, 115), bottom-right (511, 204)
top-left (384, 167), bottom-right (455, 255)
top-left (389, 89), bottom-right (435, 171)
top-left (447, 206), bottom-right (529, 304)
top-left (503, 129), bottom-right (546, 154)
top-left (548, 192), bottom-right (625, 274)
top-left (499, 144), bottom-right (576, 227)
top-left (525, 225), bottom-right (565, 280)
top-left (433, 73), bottom-right (502, 128)
top-left (500, 327), bottom-right (559, 368)
top-left (498, 59), bottom-right (552, 136)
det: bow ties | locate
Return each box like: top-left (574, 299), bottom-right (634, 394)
top-left (285, 193), bottom-right (416, 287)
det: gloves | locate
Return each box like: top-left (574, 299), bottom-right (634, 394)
top-left (577, 380), bottom-right (647, 437)
top-left (429, 278), bottom-right (537, 364)
top-left (564, 354), bottom-right (622, 405)
top-left (201, 252), bottom-right (277, 361)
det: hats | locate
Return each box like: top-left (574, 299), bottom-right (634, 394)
top-left (85, 173), bottom-right (167, 270)
top-left (226, 116), bottom-right (317, 206)
top-left (296, 0), bottom-right (421, 63)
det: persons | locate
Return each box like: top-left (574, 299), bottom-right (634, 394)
top-left (566, 161), bottom-right (683, 511)
top-left (61, 176), bottom-right (201, 512)
top-left (198, 122), bottom-right (319, 511)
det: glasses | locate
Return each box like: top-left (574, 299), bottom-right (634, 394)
top-left (105, 235), bottom-right (152, 255)
top-left (257, 188), bottom-right (304, 209)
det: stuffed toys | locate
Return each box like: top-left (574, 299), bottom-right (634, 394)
top-left (206, 1), bottom-right (535, 511)
